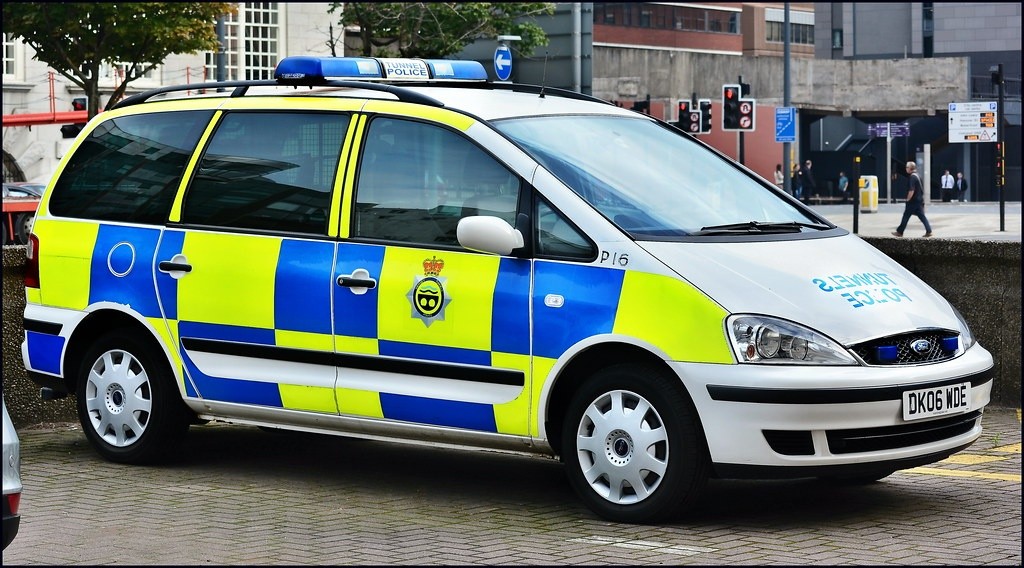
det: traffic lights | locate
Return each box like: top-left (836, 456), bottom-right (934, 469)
top-left (73, 98), bottom-right (88, 137)
top-left (698, 100), bottom-right (713, 135)
top-left (61, 125), bottom-right (73, 138)
top-left (678, 101), bottom-right (690, 132)
top-left (994, 141), bottom-right (1005, 188)
top-left (721, 84), bottom-right (741, 132)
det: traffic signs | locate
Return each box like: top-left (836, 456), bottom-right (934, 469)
top-left (947, 101), bottom-right (999, 143)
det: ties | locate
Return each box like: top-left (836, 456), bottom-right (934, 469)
top-left (945, 176), bottom-right (948, 187)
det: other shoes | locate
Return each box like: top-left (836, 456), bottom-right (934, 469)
top-left (892, 232), bottom-right (903, 237)
top-left (923, 233), bottom-right (932, 237)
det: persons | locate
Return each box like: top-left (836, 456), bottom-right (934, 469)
top-left (891, 160), bottom-right (934, 238)
top-left (941, 169), bottom-right (955, 203)
top-left (799, 159), bottom-right (818, 205)
top-left (772, 163), bottom-right (785, 191)
top-left (792, 162), bottom-right (802, 200)
top-left (954, 171), bottom-right (968, 203)
top-left (838, 170), bottom-right (849, 204)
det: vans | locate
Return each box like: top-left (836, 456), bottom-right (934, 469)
top-left (19, 53), bottom-right (995, 524)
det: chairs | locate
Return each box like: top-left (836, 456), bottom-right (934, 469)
top-left (461, 147), bottom-right (520, 229)
top-left (357, 156), bottom-right (451, 245)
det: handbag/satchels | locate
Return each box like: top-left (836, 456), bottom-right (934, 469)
top-left (911, 202), bottom-right (925, 215)
